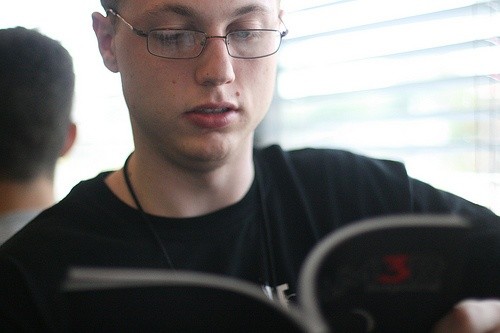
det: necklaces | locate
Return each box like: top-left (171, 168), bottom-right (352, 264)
top-left (117, 148), bottom-right (293, 322)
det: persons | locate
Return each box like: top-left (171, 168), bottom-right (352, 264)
top-left (0, 0), bottom-right (499, 333)
top-left (0, 26), bottom-right (82, 242)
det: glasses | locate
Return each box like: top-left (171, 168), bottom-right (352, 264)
top-left (105, 8), bottom-right (288, 58)
